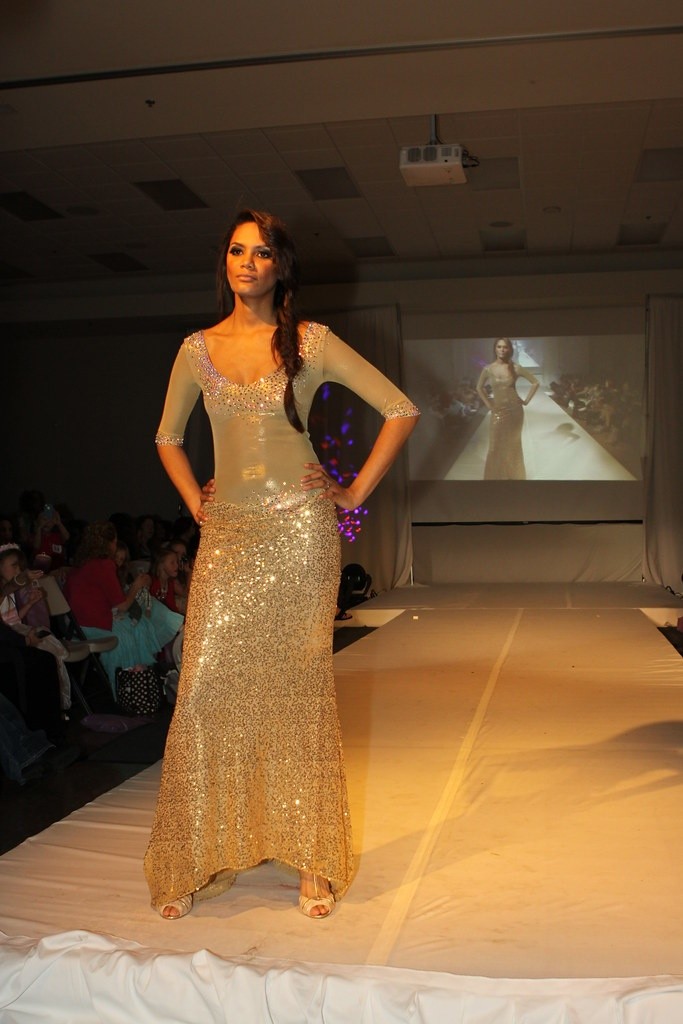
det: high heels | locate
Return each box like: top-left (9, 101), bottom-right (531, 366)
top-left (299, 869), bottom-right (334, 919)
top-left (159, 892), bottom-right (193, 919)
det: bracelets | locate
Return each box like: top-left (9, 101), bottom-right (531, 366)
top-left (14, 575), bottom-right (26, 585)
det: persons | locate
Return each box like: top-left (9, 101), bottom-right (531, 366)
top-left (559, 379), bottom-right (644, 438)
top-left (411, 376), bottom-right (487, 430)
top-left (334, 606), bottom-right (353, 621)
top-left (476, 338), bottom-right (541, 480)
top-left (142, 209), bottom-right (421, 920)
top-left (0, 491), bottom-right (200, 786)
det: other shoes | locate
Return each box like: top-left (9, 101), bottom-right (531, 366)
top-left (0, 773), bottom-right (42, 800)
top-left (22, 746), bottom-right (74, 784)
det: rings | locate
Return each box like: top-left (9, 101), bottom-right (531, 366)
top-left (327, 482), bottom-right (329, 488)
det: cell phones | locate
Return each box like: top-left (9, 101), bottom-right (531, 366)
top-left (44, 504), bottom-right (54, 520)
top-left (32, 552), bottom-right (51, 575)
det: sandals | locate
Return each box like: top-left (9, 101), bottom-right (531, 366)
top-left (334, 609), bottom-right (352, 620)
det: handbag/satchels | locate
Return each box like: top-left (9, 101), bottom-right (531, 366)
top-left (115, 665), bottom-right (166, 717)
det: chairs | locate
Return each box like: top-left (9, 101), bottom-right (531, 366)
top-left (38, 576), bottom-right (118, 717)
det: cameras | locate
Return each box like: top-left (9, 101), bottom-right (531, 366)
top-left (180, 554), bottom-right (190, 564)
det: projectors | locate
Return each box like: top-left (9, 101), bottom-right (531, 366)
top-left (398, 143), bottom-right (468, 188)
